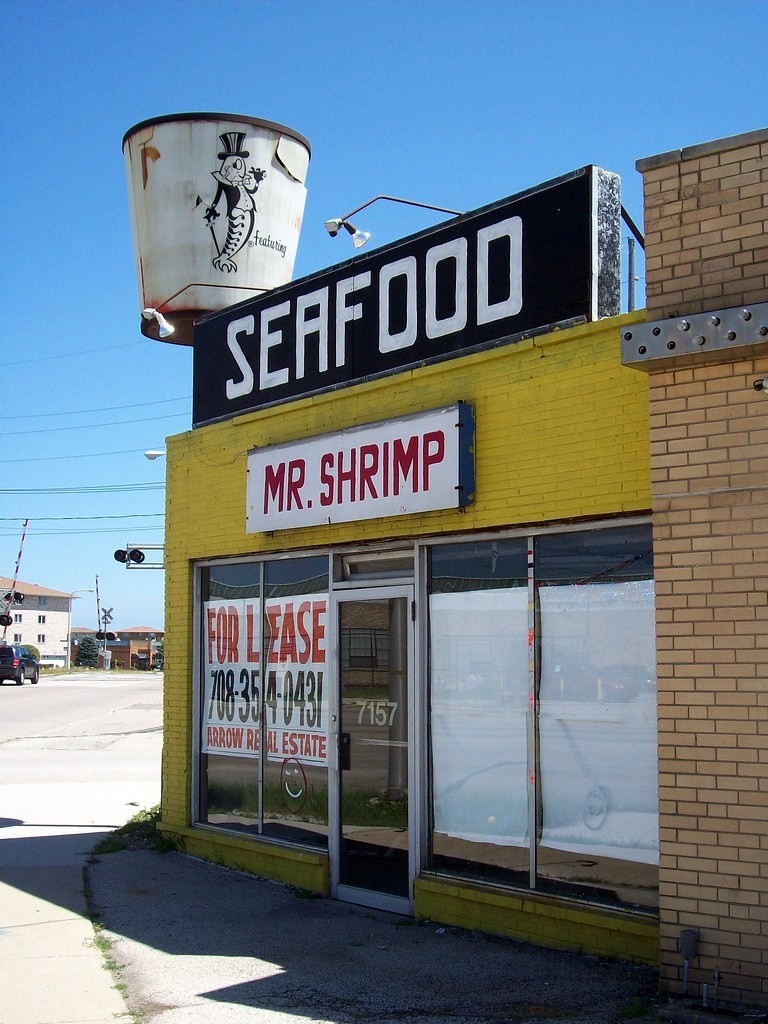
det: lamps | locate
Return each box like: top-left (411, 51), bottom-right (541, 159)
top-left (325, 194), bottom-right (465, 249)
top-left (142, 282), bottom-right (273, 337)
top-left (114, 543), bottom-right (164, 569)
top-left (5, 592), bottom-right (24, 605)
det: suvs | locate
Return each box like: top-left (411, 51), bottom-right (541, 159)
top-left (0, 644), bottom-right (40, 685)
